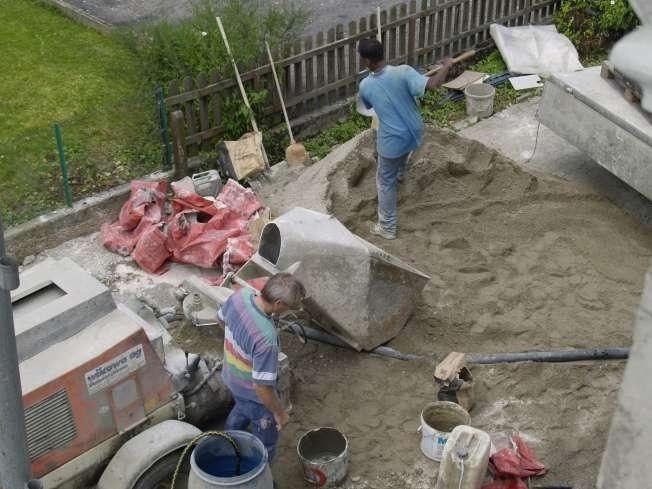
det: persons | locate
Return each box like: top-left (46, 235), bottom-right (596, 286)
top-left (212, 272), bottom-right (308, 489)
top-left (352, 36), bottom-right (458, 242)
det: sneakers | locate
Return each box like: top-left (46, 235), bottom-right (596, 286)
top-left (369, 223), bottom-right (397, 240)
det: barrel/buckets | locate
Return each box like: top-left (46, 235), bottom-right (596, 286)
top-left (438, 425), bottom-right (491, 489)
top-left (190, 430), bottom-right (273, 489)
top-left (464, 84), bottom-right (497, 118)
top-left (297, 427), bottom-right (351, 487)
top-left (419, 400), bottom-right (471, 463)
